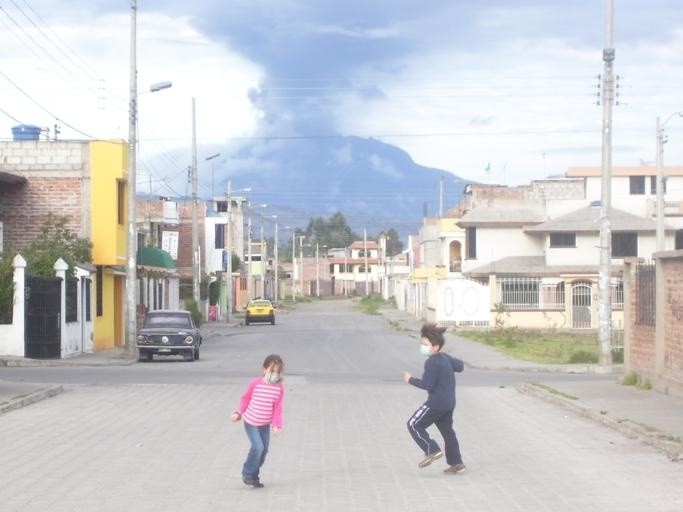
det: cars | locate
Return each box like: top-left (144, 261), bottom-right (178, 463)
top-left (241, 297), bottom-right (277, 327)
top-left (134, 309), bottom-right (204, 363)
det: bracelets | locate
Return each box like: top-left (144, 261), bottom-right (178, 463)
top-left (232, 411), bottom-right (240, 420)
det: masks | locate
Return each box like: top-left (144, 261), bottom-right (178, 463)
top-left (265, 375), bottom-right (280, 384)
top-left (419, 344), bottom-right (431, 357)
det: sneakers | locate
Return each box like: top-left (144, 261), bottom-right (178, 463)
top-left (443, 463), bottom-right (467, 474)
top-left (418, 449), bottom-right (443, 467)
top-left (242, 475), bottom-right (264, 488)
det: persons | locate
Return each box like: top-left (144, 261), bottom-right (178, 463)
top-left (231, 354), bottom-right (284, 488)
top-left (403, 324), bottom-right (465, 474)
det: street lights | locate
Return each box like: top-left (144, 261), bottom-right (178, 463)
top-left (594, 46), bottom-right (623, 374)
top-left (222, 180), bottom-right (252, 327)
top-left (121, 79), bottom-right (174, 361)
top-left (246, 199), bottom-right (329, 310)
